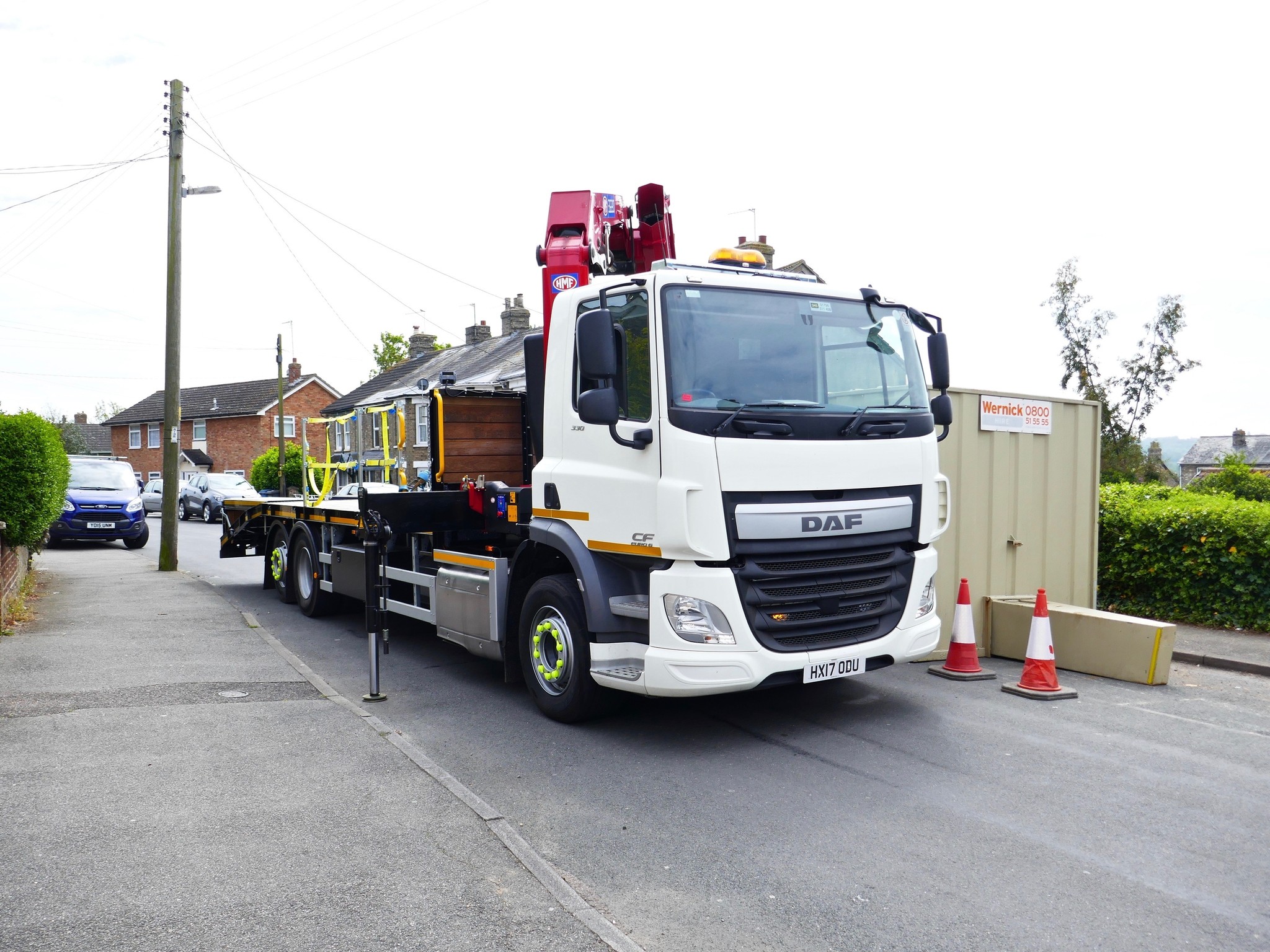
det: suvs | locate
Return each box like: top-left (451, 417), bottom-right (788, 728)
top-left (45, 455), bottom-right (150, 549)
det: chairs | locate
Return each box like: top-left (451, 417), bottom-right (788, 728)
top-left (733, 358), bottom-right (789, 404)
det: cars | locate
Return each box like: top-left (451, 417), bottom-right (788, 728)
top-left (140, 479), bottom-right (190, 517)
top-left (179, 472), bottom-right (262, 524)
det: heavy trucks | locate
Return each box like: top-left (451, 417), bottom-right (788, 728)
top-left (220, 181), bottom-right (953, 730)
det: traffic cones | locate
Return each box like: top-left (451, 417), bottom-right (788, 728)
top-left (927, 578), bottom-right (997, 681)
top-left (1000, 589), bottom-right (1079, 701)
